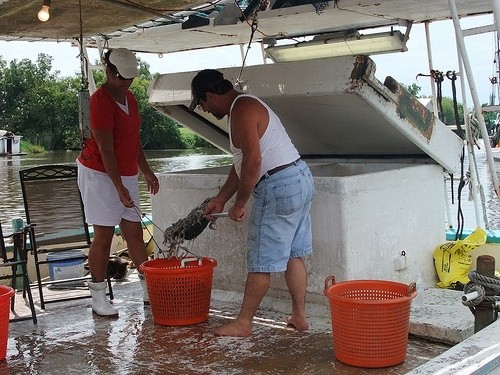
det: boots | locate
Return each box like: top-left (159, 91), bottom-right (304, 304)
top-left (87, 281), bottom-right (119, 316)
top-left (139, 272), bottom-right (150, 305)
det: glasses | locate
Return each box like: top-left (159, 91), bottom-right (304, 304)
top-left (109, 67), bottom-right (134, 80)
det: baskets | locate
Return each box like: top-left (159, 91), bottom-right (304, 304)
top-left (140, 250), bottom-right (217, 326)
top-left (324, 274), bottom-right (417, 368)
top-left (0, 283), bottom-right (15, 360)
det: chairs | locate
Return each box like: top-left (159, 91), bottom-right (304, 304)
top-left (0, 164), bottom-right (114, 324)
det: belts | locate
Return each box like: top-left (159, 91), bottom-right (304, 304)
top-left (256, 158), bottom-right (301, 188)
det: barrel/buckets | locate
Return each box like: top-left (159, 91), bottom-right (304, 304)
top-left (0, 284), bottom-right (15, 360)
top-left (46, 249), bottom-right (85, 285)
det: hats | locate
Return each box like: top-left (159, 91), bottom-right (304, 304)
top-left (109, 48), bottom-right (138, 79)
top-left (188, 69), bottom-right (223, 112)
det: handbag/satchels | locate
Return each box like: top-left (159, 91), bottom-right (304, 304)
top-left (434, 226), bottom-right (487, 289)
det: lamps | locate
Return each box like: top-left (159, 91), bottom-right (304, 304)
top-left (263, 20), bottom-right (413, 63)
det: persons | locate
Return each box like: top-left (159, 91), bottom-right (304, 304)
top-left (188, 69), bottom-right (316, 337)
top-left (75, 47), bottom-right (160, 315)
